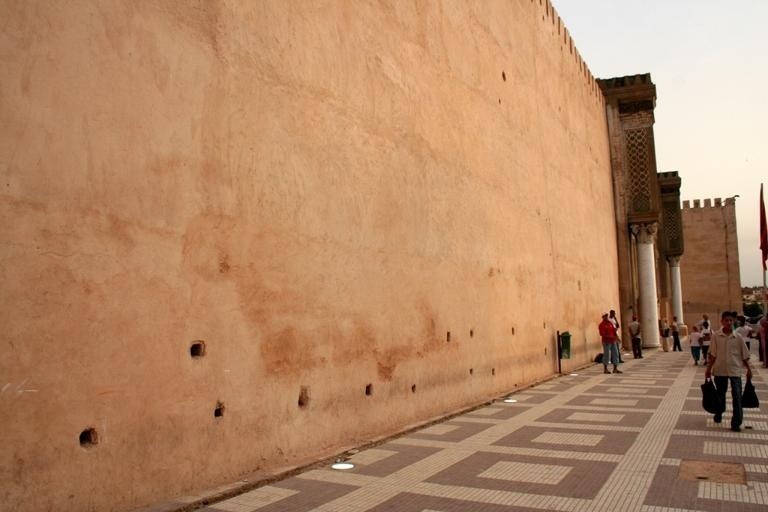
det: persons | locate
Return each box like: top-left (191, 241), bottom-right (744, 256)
top-left (690, 311), bottom-right (768, 368)
top-left (662, 317), bottom-right (683, 352)
top-left (599, 310), bottom-right (624, 373)
top-left (628, 315), bottom-right (644, 358)
top-left (705, 311), bottom-right (752, 431)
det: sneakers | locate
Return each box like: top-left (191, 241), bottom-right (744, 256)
top-left (714, 415), bottom-right (742, 432)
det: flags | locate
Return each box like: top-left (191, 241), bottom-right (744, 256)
top-left (760, 183), bottom-right (768, 271)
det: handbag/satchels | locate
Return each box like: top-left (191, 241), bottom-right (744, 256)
top-left (742, 375), bottom-right (759, 408)
top-left (701, 376), bottom-right (725, 414)
